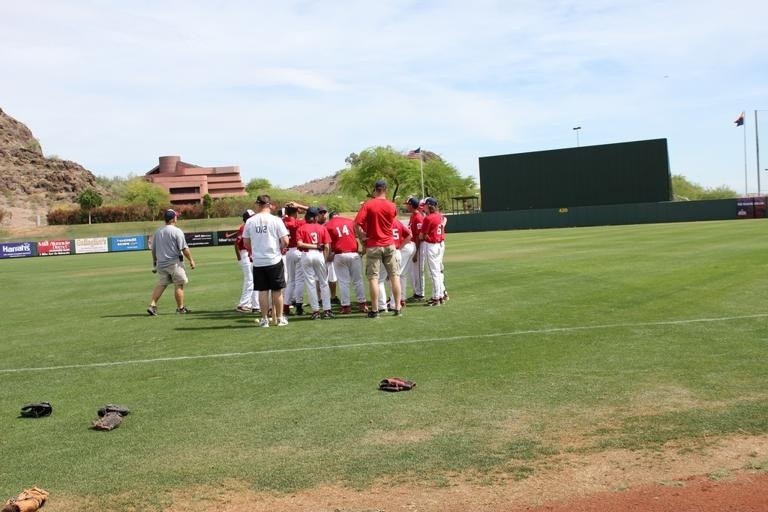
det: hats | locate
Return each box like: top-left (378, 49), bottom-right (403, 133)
top-left (329, 210), bottom-right (339, 219)
top-left (307, 207), bottom-right (318, 217)
top-left (164, 208), bottom-right (182, 222)
top-left (242, 208), bottom-right (256, 222)
top-left (278, 207), bottom-right (288, 218)
top-left (401, 197), bottom-right (437, 208)
top-left (318, 205), bottom-right (328, 214)
top-left (373, 179), bottom-right (387, 191)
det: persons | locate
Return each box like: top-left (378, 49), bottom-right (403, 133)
top-left (235, 179), bottom-right (449, 328)
top-left (147, 209), bottom-right (195, 316)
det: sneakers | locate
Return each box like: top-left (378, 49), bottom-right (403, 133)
top-left (426, 293), bottom-right (450, 307)
top-left (358, 303), bottom-right (368, 312)
top-left (235, 305), bottom-right (261, 315)
top-left (277, 316), bottom-right (288, 326)
top-left (368, 296), bottom-right (406, 317)
top-left (146, 304), bottom-right (159, 317)
top-left (331, 296), bottom-right (341, 304)
top-left (296, 310), bottom-right (306, 315)
top-left (257, 319), bottom-right (270, 328)
top-left (339, 305), bottom-right (353, 314)
top-left (175, 306), bottom-right (192, 314)
top-left (284, 310), bottom-right (295, 316)
top-left (323, 310), bottom-right (336, 319)
top-left (408, 294), bottom-right (425, 303)
top-left (309, 312), bottom-right (320, 319)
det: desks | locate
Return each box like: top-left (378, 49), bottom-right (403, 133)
top-left (449, 194), bottom-right (481, 216)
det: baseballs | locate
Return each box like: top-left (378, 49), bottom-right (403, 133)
top-left (268, 318), bottom-right (272, 321)
top-left (255, 319), bottom-right (259, 323)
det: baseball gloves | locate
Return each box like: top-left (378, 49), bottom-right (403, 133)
top-left (378, 377), bottom-right (415, 392)
top-left (21, 401), bottom-right (52, 417)
top-left (4, 486), bottom-right (48, 511)
top-left (88, 404), bottom-right (128, 429)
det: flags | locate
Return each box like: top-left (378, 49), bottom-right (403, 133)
top-left (734, 111), bottom-right (744, 127)
top-left (414, 147), bottom-right (421, 154)
